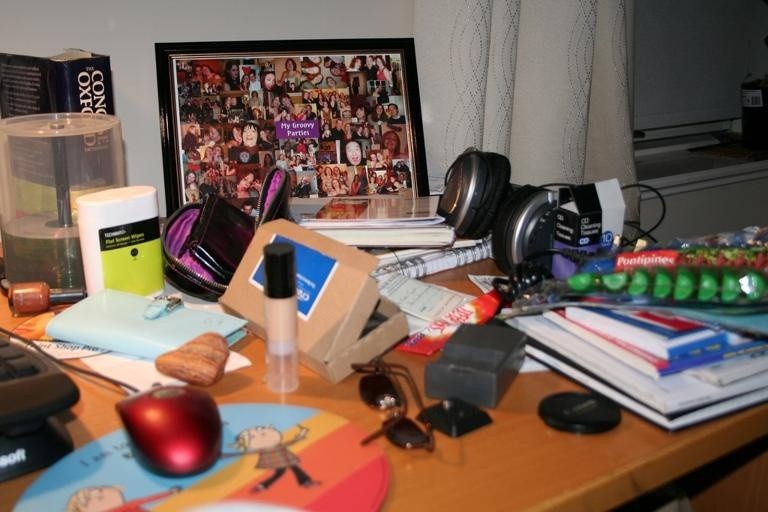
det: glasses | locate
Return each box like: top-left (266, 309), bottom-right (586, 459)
top-left (352, 360), bottom-right (436, 452)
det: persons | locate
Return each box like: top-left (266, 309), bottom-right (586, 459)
top-left (177, 56), bottom-right (412, 217)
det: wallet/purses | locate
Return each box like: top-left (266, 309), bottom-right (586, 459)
top-left (190, 192), bottom-right (253, 286)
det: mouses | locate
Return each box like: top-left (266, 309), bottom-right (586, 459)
top-left (114, 384), bottom-right (223, 477)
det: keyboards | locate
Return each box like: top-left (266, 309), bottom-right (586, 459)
top-left (1, 329), bottom-right (80, 425)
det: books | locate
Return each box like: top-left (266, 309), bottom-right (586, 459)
top-left (302, 217), bottom-right (491, 285)
top-left (1, 49), bottom-right (114, 223)
top-left (503, 308), bottom-right (768, 434)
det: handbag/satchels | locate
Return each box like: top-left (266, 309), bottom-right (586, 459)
top-left (161, 166), bottom-right (289, 302)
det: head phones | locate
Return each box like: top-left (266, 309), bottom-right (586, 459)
top-left (438, 149), bottom-right (558, 274)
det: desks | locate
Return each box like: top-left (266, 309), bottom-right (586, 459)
top-left (0, 257), bottom-right (768, 512)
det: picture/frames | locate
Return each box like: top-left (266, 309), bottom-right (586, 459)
top-left (154, 38), bottom-right (431, 221)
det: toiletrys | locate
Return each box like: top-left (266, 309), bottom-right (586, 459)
top-left (8, 282), bottom-right (86, 317)
top-left (263, 242), bottom-right (298, 390)
